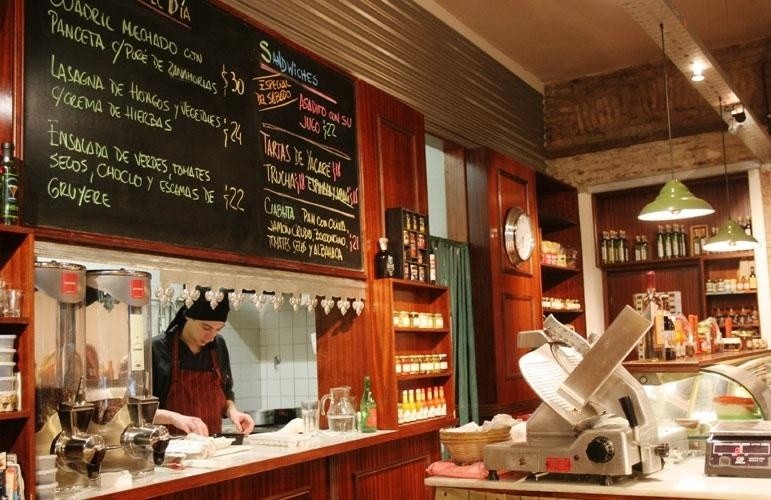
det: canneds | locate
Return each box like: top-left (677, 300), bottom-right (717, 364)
top-left (393, 310), bottom-right (444, 329)
top-left (394, 350), bottom-right (449, 375)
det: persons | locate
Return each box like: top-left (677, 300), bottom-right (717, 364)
top-left (127, 285), bottom-right (255, 439)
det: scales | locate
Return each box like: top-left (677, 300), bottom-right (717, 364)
top-left (701, 418), bottom-right (771, 477)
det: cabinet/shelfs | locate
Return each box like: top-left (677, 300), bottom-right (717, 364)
top-left (589, 169), bottom-right (771, 356)
top-left (0, 221), bottom-right (36, 500)
top-left (538, 165), bottom-right (587, 343)
top-left (374, 274), bottom-right (454, 428)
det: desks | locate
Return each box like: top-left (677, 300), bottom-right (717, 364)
top-left (425, 429), bottom-right (771, 499)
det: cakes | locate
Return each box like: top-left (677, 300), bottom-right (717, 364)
top-left (714, 394), bottom-right (755, 417)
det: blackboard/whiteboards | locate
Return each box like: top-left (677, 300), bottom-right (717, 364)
top-left (13, 0), bottom-right (369, 282)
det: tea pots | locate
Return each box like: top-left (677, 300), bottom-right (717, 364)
top-left (320, 385), bottom-right (357, 433)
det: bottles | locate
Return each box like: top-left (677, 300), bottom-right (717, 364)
top-left (661, 295), bottom-right (676, 360)
top-left (376, 238), bottom-right (395, 278)
top-left (429, 238), bottom-right (437, 283)
top-left (670, 306), bottom-right (681, 358)
top-left (708, 302), bottom-right (759, 324)
top-left (706, 265), bottom-right (757, 292)
top-left (676, 312), bottom-right (686, 356)
top-left (397, 386), bottom-right (446, 424)
top-left (599, 216), bottom-right (758, 262)
top-left (643, 271), bottom-right (667, 362)
top-left (360, 376), bottom-right (377, 433)
top-left (542, 296), bottom-right (581, 310)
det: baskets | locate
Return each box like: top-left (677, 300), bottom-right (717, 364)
top-left (438, 426), bottom-right (515, 465)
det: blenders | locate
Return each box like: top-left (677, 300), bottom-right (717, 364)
top-left (34, 256), bottom-right (106, 480)
top-left (83, 269), bottom-right (170, 477)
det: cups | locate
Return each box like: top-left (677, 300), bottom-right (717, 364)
top-left (1, 282), bottom-right (22, 317)
top-left (301, 400), bottom-right (319, 433)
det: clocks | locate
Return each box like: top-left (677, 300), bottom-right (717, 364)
top-left (505, 206), bottom-right (537, 267)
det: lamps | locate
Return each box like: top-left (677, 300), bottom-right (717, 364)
top-left (637, 20), bottom-right (715, 222)
top-left (702, 96), bottom-right (761, 251)
